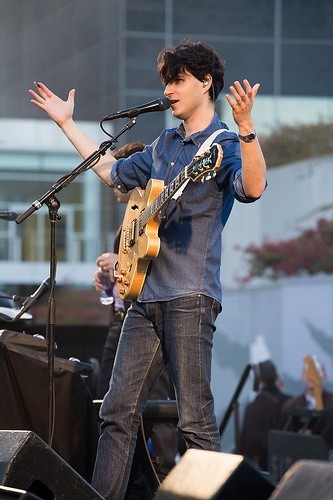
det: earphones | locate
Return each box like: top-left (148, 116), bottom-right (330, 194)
top-left (203, 79), bottom-right (209, 85)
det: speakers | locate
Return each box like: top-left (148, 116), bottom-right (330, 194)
top-left (155, 447), bottom-right (333, 500)
top-left (0, 429), bottom-right (105, 500)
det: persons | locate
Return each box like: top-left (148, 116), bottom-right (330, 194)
top-left (28, 39), bottom-right (269, 500)
top-left (281, 363), bottom-right (333, 432)
top-left (243, 360), bottom-right (296, 430)
top-left (94, 141), bottom-right (181, 479)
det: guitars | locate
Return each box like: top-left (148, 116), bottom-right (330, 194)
top-left (303, 353), bottom-right (326, 412)
top-left (117, 143), bottom-right (224, 303)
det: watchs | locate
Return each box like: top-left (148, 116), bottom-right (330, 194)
top-left (239, 131), bottom-right (256, 143)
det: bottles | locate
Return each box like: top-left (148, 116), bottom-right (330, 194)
top-left (98, 266), bottom-right (114, 305)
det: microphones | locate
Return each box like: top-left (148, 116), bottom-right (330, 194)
top-left (13, 278), bottom-right (56, 321)
top-left (103, 97), bottom-right (171, 121)
